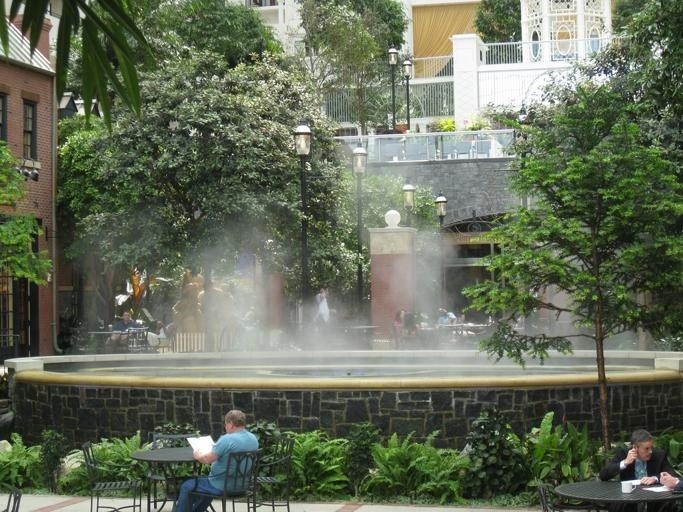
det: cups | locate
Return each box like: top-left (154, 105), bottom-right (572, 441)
top-left (108, 325), bottom-right (112, 332)
top-left (621, 482), bottom-right (636, 494)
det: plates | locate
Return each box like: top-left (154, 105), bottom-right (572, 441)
top-left (628, 480), bottom-right (641, 486)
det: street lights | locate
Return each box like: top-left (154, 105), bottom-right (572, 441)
top-left (350, 138), bottom-right (367, 350)
top-left (402, 55), bottom-right (412, 129)
top-left (402, 178), bottom-right (415, 226)
top-left (519, 104), bottom-right (529, 209)
top-left (435, 191), bottom-right (448, 307)
top-left (291, 114), bottom-right (314, 349)
top-left (388, 42), bottom-right (398, 133)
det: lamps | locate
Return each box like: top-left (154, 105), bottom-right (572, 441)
top-left (15, 155), bottom-right (39, 182)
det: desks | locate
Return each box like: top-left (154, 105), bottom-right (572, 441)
top-left (87, 329), bottom-right (134, 353)
top-left (553, 478), bottom-right (682, 511)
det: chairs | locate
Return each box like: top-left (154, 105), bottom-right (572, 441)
top-left (535, 475), bottom-right (601, 511)
top-left (82, 434), bottom-right (294, 512)
top-left (126, 326), bottom-right (148, 352)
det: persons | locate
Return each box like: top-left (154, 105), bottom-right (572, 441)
top-left (174, 409), bottom-right (259, 512)
top-left (312, 286), bottom-right (329, 325)
top-left (111, 311), bottom-right (139, 341)
top-left (659, 471), bottom-right (683, 491)
top-left (392, 307), bottom-right (466, 336)
top-left (599, 429), bottom-right (681, 486)
